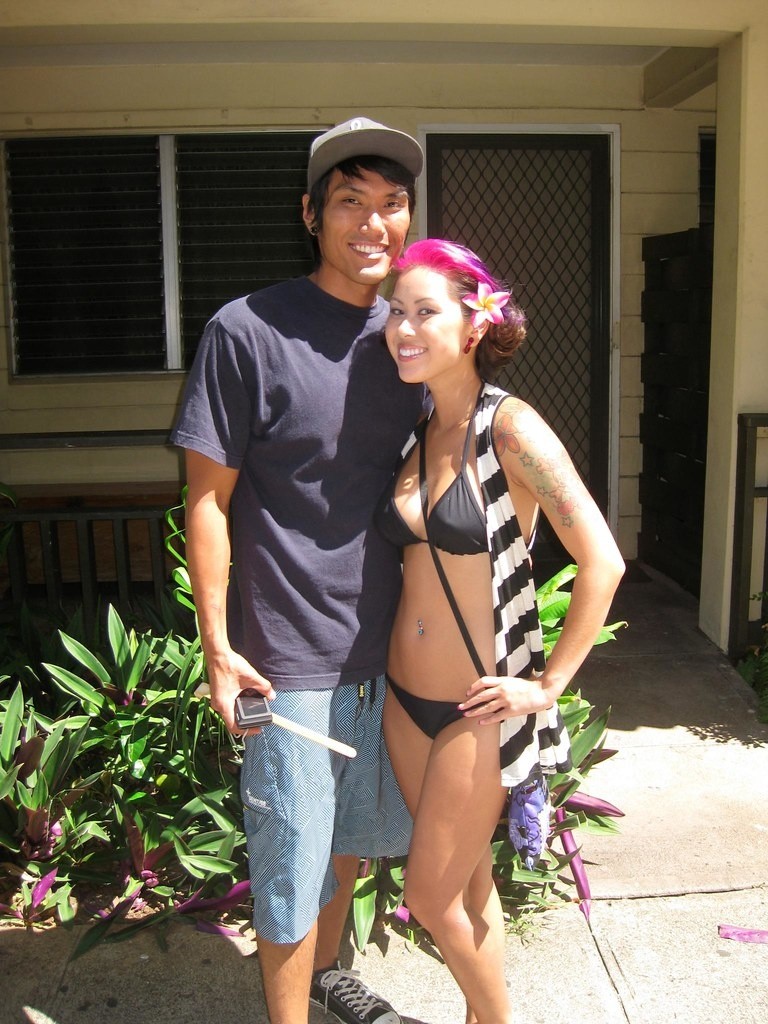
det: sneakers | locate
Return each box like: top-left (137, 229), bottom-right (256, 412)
top-left (309, 958), bottom-right (403, 1024)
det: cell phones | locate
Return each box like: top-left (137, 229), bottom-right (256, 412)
top-left (235, 688), bottom-right (272, 730)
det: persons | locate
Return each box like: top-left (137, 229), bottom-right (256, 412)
top-left (380, 239), bottom-right (627, 1024)
top-left (169, 117), bottom-right (424, 1024)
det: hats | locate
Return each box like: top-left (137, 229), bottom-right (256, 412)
top-left (307, 116), bottom-right (424, 194)
